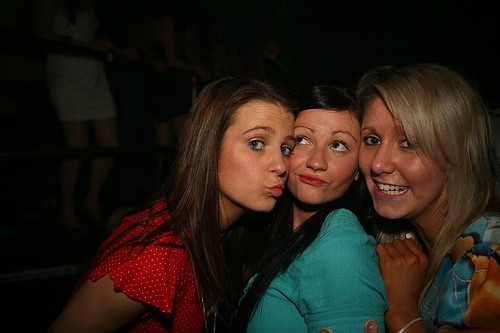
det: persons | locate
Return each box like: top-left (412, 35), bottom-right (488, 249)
top-left (141, 10), bottom-right (213, 147)
top-left (221, 82), bottom-right (390, 333)
top-left (350, 64), bottom-right (500, 333)
top-left (48, 75), bottom-right (296, 333)
top-left (37, 1), bottom-right (122, 231)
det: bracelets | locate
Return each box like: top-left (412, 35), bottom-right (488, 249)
top-left (396, 317), bottom-right (424, 333)
